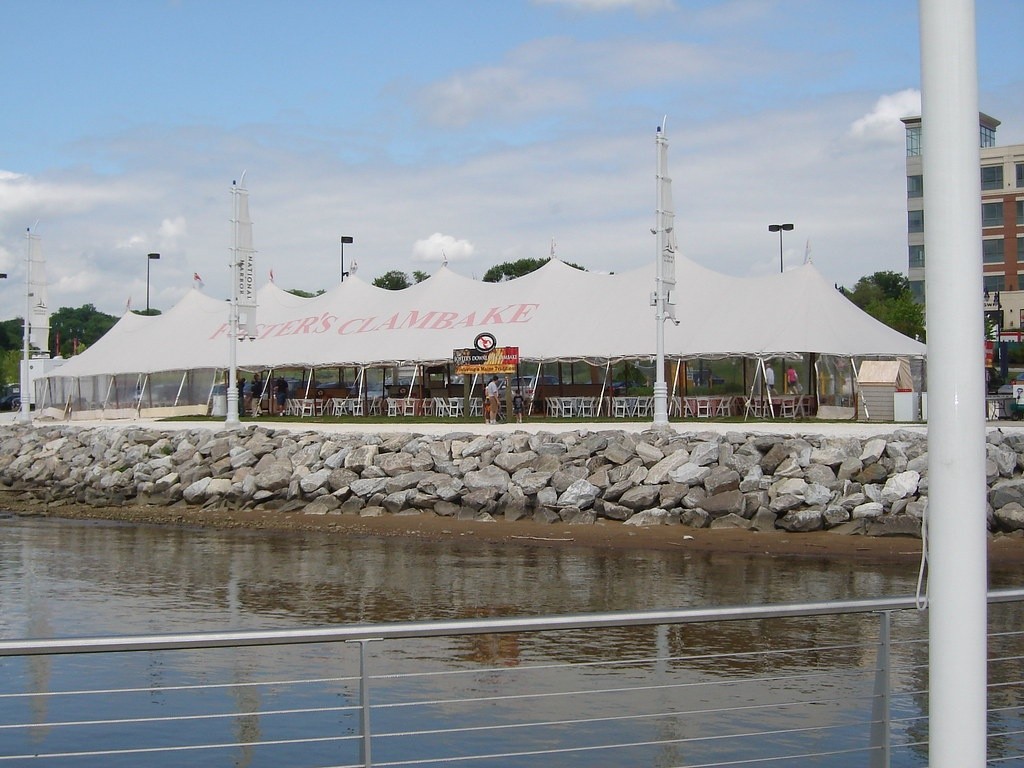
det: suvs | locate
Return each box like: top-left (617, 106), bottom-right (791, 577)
top-left (445, 375), bottom-right (570, 413)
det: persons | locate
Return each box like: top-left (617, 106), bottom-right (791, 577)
top-left (1010, 378), bottom-right (1016, 385)
top-left (623, 363), bottom-right (634, 383)
top-left (277, 375), bottom-right (289, 417)
top-left (787, 364), bottom-right (800, 395)
top-left (484, 381), bottom-right (492, 424)
top-left (487, 375), bottom-right (501, 425)
top-left (481, 383), bottom-right (487, 420)
top-left (237, 378), bottom-right (246, 417)
top-left (764, 363), bottom-right (779, 396)
top-left (513, 390), bottom-right (524, 423)
top-left (250, 374), bottom-right (263, 418)
top-left (630, 364), bottom-right (650, 388)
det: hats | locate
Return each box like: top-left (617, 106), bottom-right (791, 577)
top-left (280, 376), bottom-right (284, 379)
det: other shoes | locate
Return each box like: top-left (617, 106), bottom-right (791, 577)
top-left (519, 421), bottom-right (522, 424)
top-left (517, 419), bottom-right (519, 422)
top-left (490, 422), bottom-right (500, 425)
top-left (251, 413), bottom-right (261, 417)
top-left (279, 409), bottom-right (286, 417)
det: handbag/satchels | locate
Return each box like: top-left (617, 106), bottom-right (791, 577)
top-left (796, 383), bottom-right (803, 392)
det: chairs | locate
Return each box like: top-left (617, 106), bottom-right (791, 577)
top-left (716, 396), bottom-right (732, 416)
top-left (754, 397), bottom-right (770, 418)
top-left (696, 396), bottom-right (712, 418)
top-left (674, 397), bottom-right (694, 418)
top-left (743, 397), bottom-right (755, 416)
top-left (779, 397), bottom-right (795, 418)
top-left (387, 397), bottom-right (482, 418)
top-left (795, 394), bottom-right (805, 417)
top-left (286, 398), bottom-right (363, 417)
top-left (368, 397), bottom-right (383, 416)
top-left (545, 396), bottom-right (653, 418)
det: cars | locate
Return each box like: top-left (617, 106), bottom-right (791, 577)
top-left (585, 382), bottom-right (647, 398)
top-left (132, 381), bottom-right (229, 401)
top-left (253, 378), bottom-right (410, 399)
top-left (985, 367), bottom-right (1005, 394)
top-left (0, 382), bottom-right (21, 412)
top-left (686, 370), bottom-right (725, 385)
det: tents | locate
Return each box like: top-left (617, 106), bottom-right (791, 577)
top-left (29, 249), bottom-right (927, 430)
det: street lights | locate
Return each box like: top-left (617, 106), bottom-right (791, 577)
top-left (147, 253), bottom-right (160, 310)
top-left (984, 285), bottom-right (1001, 366)
top-left (341, 236), bottom-right (353, 284)
top-left (768, 224), bottom-right (794, 272)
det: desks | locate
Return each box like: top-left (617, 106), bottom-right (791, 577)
top-left (985, 397), bottom-right (1014, 421)
